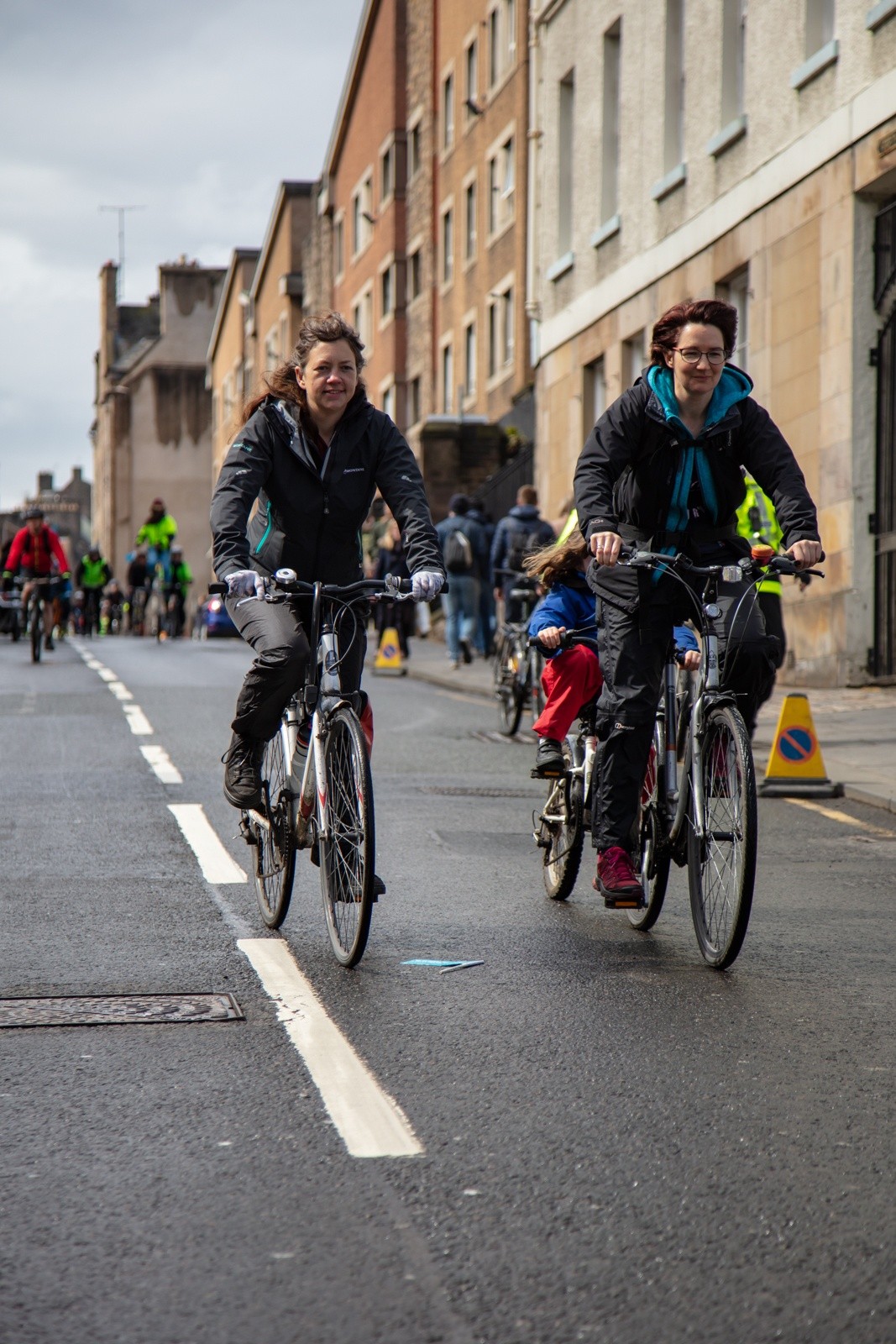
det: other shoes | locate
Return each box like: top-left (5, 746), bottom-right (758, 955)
top-left (459, 636), bottom-right (474, 664)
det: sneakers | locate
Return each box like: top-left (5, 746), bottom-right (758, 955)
top-left (596, 847), bottom-right (646, 901)
top-left (221, 731), bottom-right (268, 810)
top-left (536, 738), bottom-right (566, 773)
top-left (344, 853), bottom-right (386, 894)
top-left (708, 731), bottom-right (742, 791)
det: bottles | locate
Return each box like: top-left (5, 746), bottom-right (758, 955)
top-left (290, 722), bottom-right (314, 793)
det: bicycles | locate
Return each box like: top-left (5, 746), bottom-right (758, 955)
top-left (205, 571), bottom-right (449, 971)
top-left (0, 558), bottom-right (193, 667)
top-left (585, 540), bottom-right (826, 970)
top-left (488, 566), bottom-right (552, 743)
top-left (522, 628), bottom-right (689, 901)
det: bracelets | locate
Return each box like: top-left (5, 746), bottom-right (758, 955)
top-left (2, 571), bottom-right (12, 578)
top-left (61, 572), bottom-right (71, 578)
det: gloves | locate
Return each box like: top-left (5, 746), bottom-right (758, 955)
top-left (225, 570), bottom-right (265, 602)
top-left (410, 571), bottom-right (445, 603)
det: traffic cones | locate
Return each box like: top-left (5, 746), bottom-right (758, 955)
top-left (754, 692), bottom-right (841, 800)
top-left (370, 627), bottom-right (409, 677)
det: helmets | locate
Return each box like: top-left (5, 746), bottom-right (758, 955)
top-left (23, 506), bottom-right (45, 521)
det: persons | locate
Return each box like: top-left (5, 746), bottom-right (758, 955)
top-left (466, 496), bottom-right (500, 658)
top-left (135, 499), bottom-right (178, 590)
top-left (736, 475), bottom-right (811, 671)
top-left (574, 302), bottom-right (821, 897)
top-left (75, 578), bottom-right (123, 635)
top-left (0, 538), bottom-right (71, 633)
top-left (490, 485), bottom-right (556, 624)
top-left (210, 315), bottom-right (447, 893)
top-left (366, 502), bottom-right (417, 658)
top-left (434, 493), bottom-right (487, 670)
top-left (520, 528), bottom-right (701, 812)
top-left (125, 543), bottom-right (209, 640)
top-left (75, 547), bottom-right (112, 637)
top-left (3, 509), bottom-right (71, 649)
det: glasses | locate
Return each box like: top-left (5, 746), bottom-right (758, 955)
top-left (671, 347), bottom-right (728, 364)
